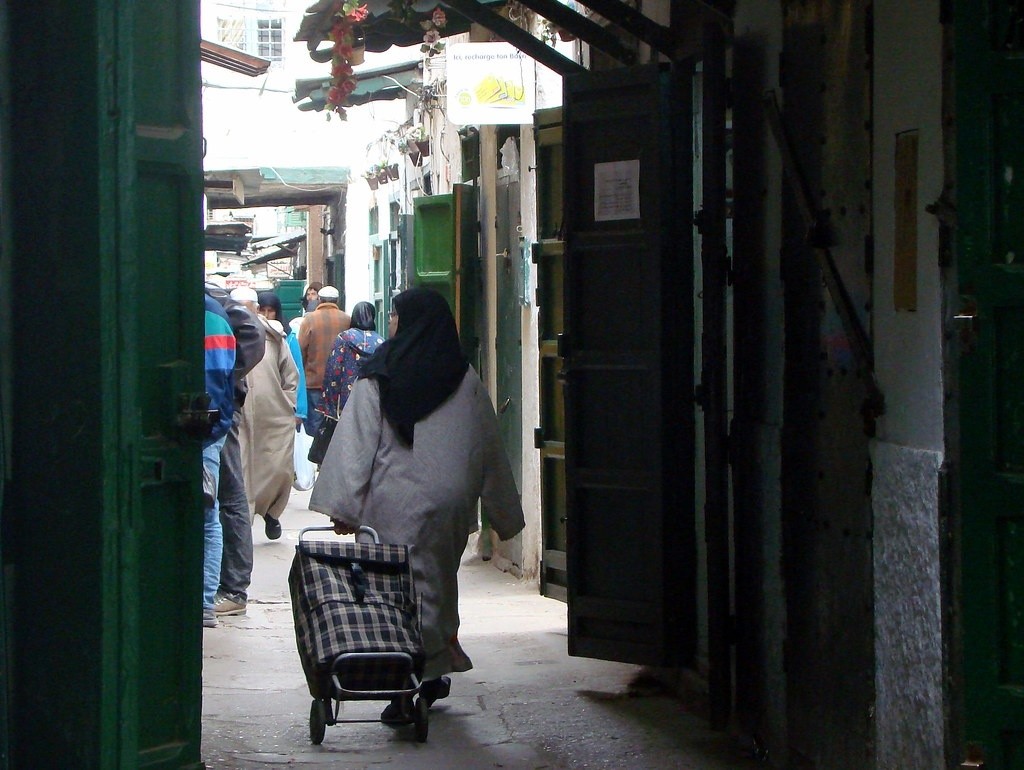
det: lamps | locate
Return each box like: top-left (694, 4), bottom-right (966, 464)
top-left (320, 228), bottom-right (334, 235)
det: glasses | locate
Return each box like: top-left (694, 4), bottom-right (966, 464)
top-left (387, 310), bottom-right (397, 321)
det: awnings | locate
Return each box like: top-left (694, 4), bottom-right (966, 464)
top-left (291, 62), bottom-right (421, 112)
top-left (199, 41), bottom-right (271, 76)
top-left (244, 230), bottom-right (306, 276)
top-left (295, 0), bottom-right (677, 75)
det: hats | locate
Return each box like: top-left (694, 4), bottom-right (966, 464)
top-left (230, 287), bottom-right (258, 302)
top-left (318, 286), bottom-right (339, 300)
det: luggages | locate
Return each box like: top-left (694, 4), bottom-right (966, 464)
top-left (288, 524), bottom-right (429, 743)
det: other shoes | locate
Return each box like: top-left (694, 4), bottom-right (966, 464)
top-left (203, 609), bottom-right (218, 627)
top-left (420, 677), bottom-right (451, 702)
top-left (263, 514), bottom-right (281, 540)
top-left (381, 697), bottom-right (415, 724)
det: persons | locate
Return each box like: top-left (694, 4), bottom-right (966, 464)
top-left (203, 279), bottom-right (324, 627)
top-left (298, 286), bottom-right (351, 436)
top-left (308, 288), bottom-right (525, 726)
top-left (314, 302), bottom-right (385, 421)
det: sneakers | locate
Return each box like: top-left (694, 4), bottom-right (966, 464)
top-left (214, 591), bottom-right (247, 616)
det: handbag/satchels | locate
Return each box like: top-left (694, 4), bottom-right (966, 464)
top-left (308, 417), bottom-right (338, 465)
top-left (293, 422), bottom-right (316, 491)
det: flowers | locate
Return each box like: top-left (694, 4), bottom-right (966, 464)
top-left (420, 7), bottom-right (449, 56)
top-left (323, 0), bottom-right (368, 123)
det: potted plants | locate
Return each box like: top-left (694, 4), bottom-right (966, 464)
top-left (365, 162), bottom-right (399, 192)
top-left (540, 18), bottom-right (575, 45)
top-left (397, 128), bottom-right (430, 167)
